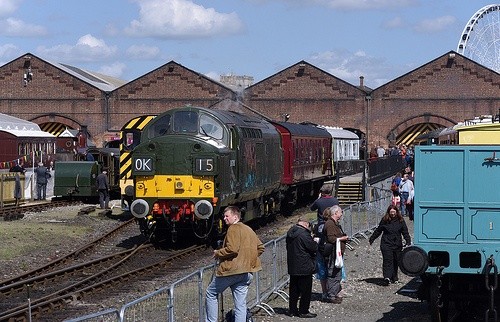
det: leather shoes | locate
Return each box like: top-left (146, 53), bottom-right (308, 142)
top-left (300, 311), bottom-right (317, 317)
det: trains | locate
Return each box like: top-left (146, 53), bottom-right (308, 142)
top-left (54, 146), bottom-right (119, 199)
top-left (127, 105), bottom-right (360, 246)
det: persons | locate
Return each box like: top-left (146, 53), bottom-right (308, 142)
top-left (369, 204), bottom-right (411, 285)
top-left (47, 161), bottom-right (55, 170)
top-left (369, 141), bottom-right (386, 160)
top-left (13, 161), bottom-right (27, 174)
top-left (391, 167), bottom-right (415, 221)
top-left (205, 207), bottom-right (265, 322)
top-left (286, 217), bottom-right (319, 318)
top-left (96, 171), bottom-right (111, 209)
top-left (83, 151), bottom-right (94, 161)
top-left (385, 143), bottom-right (415, 162)
top-left (34, 162), bottom-right (51, 200)
top-left (314, 204), bottom-right (349, 304)
top-left (309, 184), bottom-right (339, 225)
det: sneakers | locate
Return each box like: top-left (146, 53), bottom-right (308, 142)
top-left (384, 277), bottom-right (391, 286)
